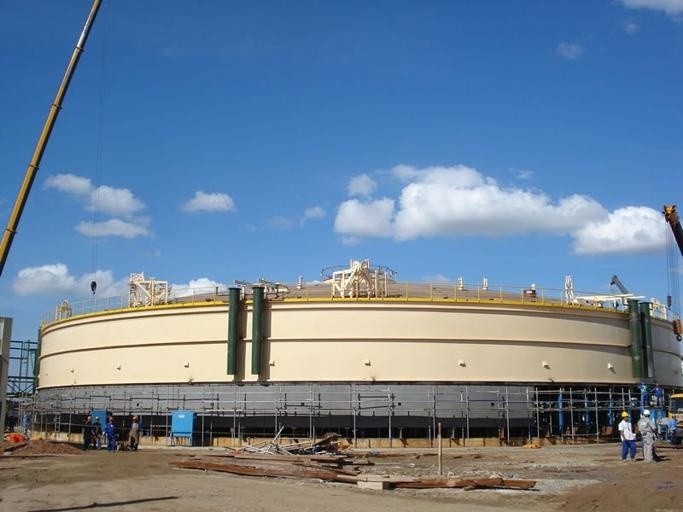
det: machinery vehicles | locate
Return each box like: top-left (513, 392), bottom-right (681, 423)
top-left (660, 200), bottom-right (683, 445)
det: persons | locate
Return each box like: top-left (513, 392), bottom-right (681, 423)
top-left (22, 414), bottom-right (32, 438)
top-left (617, 410), bottom-right (637, 462)
top-left (8, 415), bottom-right (17, 434)
top-left (90, 417), bottom-right (103, 450)
top-left (127, 414), bottom-right (140, 452)
top-left (638, 409), bottom-right (657, 463)
top-left (83, 415), bottom-right (92, 450)
top-left (102, 415), bottom-right (118, 452)
top-left (669, 413), bottom-right (680, 444)
top-left (636, 412), bottom-right (659, 458)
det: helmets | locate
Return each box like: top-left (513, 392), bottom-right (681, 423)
top-left (620, 411), bottom-right (629, 417)
top-left (643, 409), bottom-right (651, 415)
